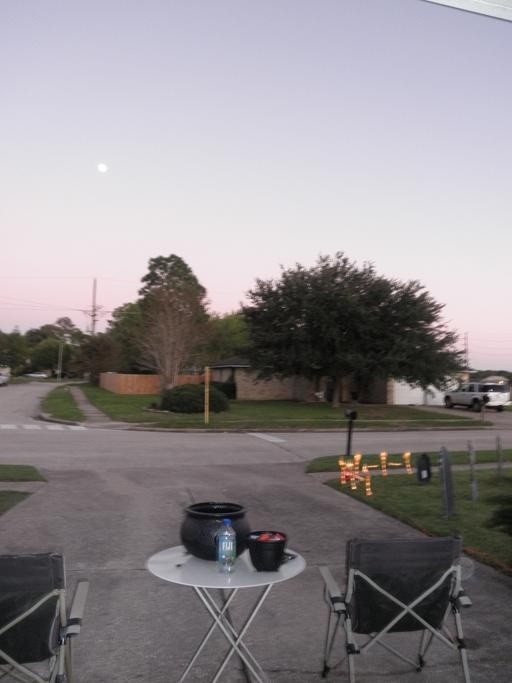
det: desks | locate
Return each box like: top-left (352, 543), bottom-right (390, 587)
top-left (146, 544), bottom-right (307, 682)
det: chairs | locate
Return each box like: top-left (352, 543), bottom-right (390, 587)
top-left (0, 547), bottom-right (90, 682)
top-left (316, 534), bottom-right (476, 682)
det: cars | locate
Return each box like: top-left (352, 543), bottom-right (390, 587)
top-left (24, 367), bottom-right (76, 379)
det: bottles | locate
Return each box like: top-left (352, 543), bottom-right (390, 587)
top-left (218, 520), bottom-right (236, 575)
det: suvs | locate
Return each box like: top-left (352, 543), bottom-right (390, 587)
top-left (445, 380), bottom-right (512, 411)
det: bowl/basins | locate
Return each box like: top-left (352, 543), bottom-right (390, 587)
top-left (180, 501), bottom-right (251, 563)
top-left (245, 531), bottom-right (287, 572)
